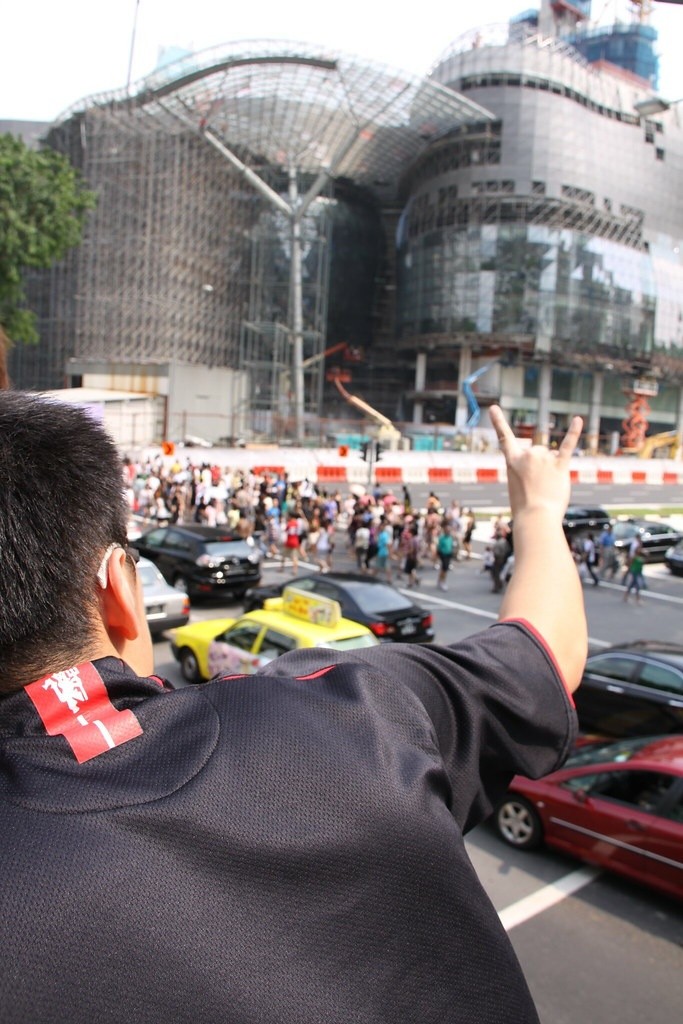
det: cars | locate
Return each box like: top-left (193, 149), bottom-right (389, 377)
top-left (587, 519), bottom-right (681, 565)
top-left (115, 555), bottom-right (190, 640)
top-left (245, 571), bottom-right (437, 652)
top-left (170, 586), bottom-right (381, 683)
top-left (663, 542), bottom-right (683, 576)
top-left (494, 734), bottom-right (682, 906)
top-left (566, 641), bottom-right (683, 740)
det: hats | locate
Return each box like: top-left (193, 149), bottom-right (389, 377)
top-left (641, 547), bottom-right (648, 556)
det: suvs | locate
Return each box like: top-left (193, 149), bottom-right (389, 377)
top-left (126, 524), bottom-right (262, 602)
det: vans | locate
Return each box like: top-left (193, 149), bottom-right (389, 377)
top-left (503, 502), bottom-right (611, 536)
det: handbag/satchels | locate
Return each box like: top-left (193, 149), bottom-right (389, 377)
top-left (354, 539), bottom-right (364, 548)
top-left (593, 552), bottom-right (600, 566)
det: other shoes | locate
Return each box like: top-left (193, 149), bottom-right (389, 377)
top-left (416, 578), bottom-right (420, 586)
top-left (321, 565), bottom-right (329, 574)
top-left (407, 583), bottom-right (412, 588)
top-left (437, 583), bottom-right (448, 590)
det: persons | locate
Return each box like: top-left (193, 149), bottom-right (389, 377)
top-left (119, 432), bottom-right (653, 607)
top-left (0, 395), bottom-right (589, 1024)
